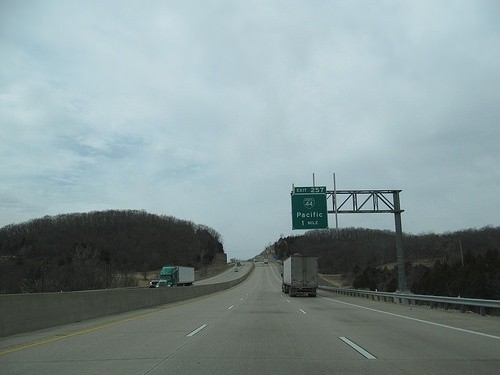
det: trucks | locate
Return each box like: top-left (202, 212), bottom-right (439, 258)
top-left (264, 259), bottom-right (268, 264)
top-left (158, 266), bottom-right (195, 286)
top-left (281, 255), bottom-right (319, 297)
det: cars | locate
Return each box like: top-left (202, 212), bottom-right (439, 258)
top-left (234, 268), bottom-right (238, 272)
top-left (149, 278), bottom-right (160, 288)
